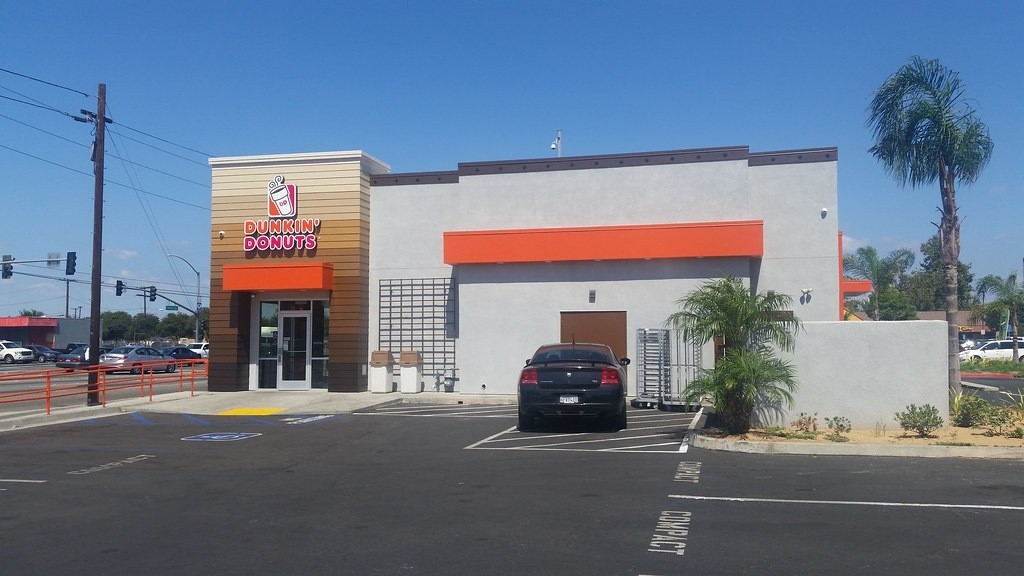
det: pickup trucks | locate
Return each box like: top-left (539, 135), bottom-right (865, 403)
top-left (52, 342), bottom-right (92, 354)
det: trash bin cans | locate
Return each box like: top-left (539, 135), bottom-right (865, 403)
top-left (370, 351), bottom-right (395, 394)
top-left (400, 351), bottom-right (421, 391)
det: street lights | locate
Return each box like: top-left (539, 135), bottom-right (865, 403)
top-left (550, 128), bottom-right (563, 159)
top-left (164, 253), bottom-right (200, 344)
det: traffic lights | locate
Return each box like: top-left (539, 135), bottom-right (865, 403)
top-left (115, 279), bottom-right (124, 297)
top-left (149, 285), bottom-right (157, 302)
top-left (65, 251), bottom-right (77, 275)
top-left (2, 254), bottom-right (14, 279)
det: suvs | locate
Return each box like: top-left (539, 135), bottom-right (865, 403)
top-left (959, 337), bottom-right (1024, 364)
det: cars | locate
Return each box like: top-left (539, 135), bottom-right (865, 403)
top-left (152, 345), bottom-right (202, 367)
top-left (0, 340), bottom-right (35, 364)
top-left (99, 345), bottom-right (178, 375)
top-left (26, 344), bottom-right (61, 363)
top-left (517, 342), bottom-right (630, 433)
top-left (185, 343), bottom-right (210, 359)
top-left (55, 347), bottom-right (115, 373)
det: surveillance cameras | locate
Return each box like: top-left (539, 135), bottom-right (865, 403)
top-left (801, 289), bottom-right (813, 294)
top-left (551, 144), bottom-right (556, 151)
